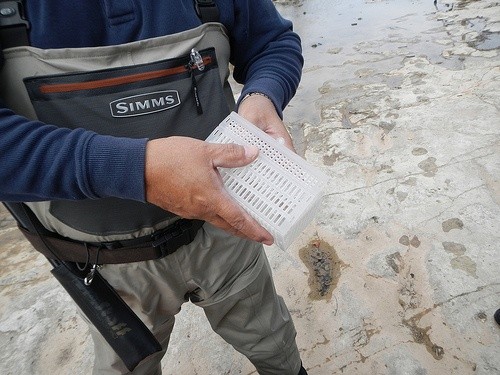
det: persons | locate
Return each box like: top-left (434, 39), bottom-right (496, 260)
top-left (0, 0), bottom-right (308, 375)
